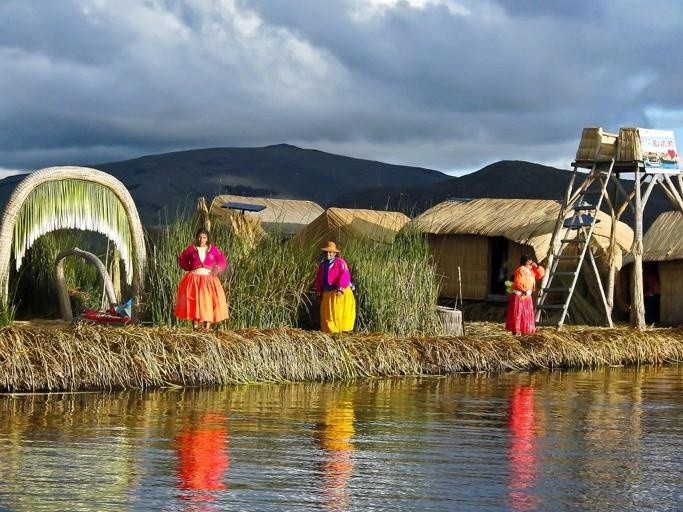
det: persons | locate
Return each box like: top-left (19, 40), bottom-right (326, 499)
top-left (503, 253), bottom-right (546, 335)
top-left (643, 264), bottom-right (659, 324)
top-left (315, 240), bottom-right (357, 334)
top-left (174, 227), bottom-right (230, 334)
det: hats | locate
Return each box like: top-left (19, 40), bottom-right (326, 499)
top-left (320, 241), bottom-right (340, 252)
